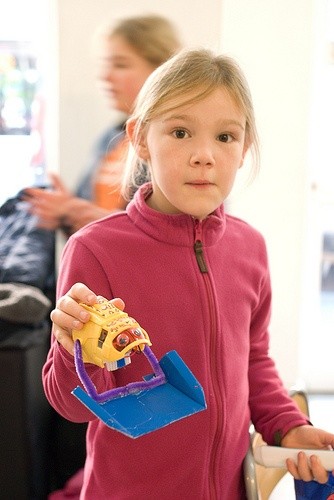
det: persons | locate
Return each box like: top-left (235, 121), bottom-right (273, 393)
top-left (16, 14), bottom-right (188, 238)
top-left (40, 48), bottom-right (334, 500)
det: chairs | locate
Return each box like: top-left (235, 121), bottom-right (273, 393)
top-left (243, 392), bottom-right (334, 500)
